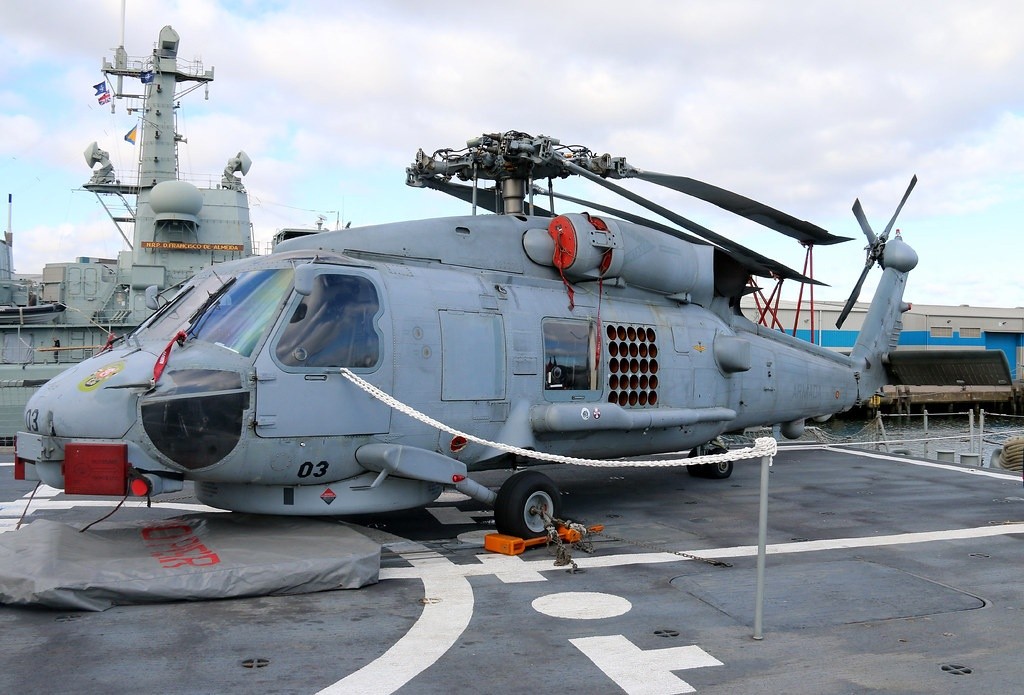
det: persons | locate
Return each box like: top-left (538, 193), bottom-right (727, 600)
top-left (53, 337), bottom-right (61, 363)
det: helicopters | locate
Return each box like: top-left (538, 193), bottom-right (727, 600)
top-left (12, 131), bottom-right (920, 540)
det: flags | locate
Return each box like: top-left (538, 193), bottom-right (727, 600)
top-left (98, 90), bottom-right (112, 106)
top-left (93, 81), bottom-right (106, 96)
top-left (140, 70), bottom-right (153, 83)
